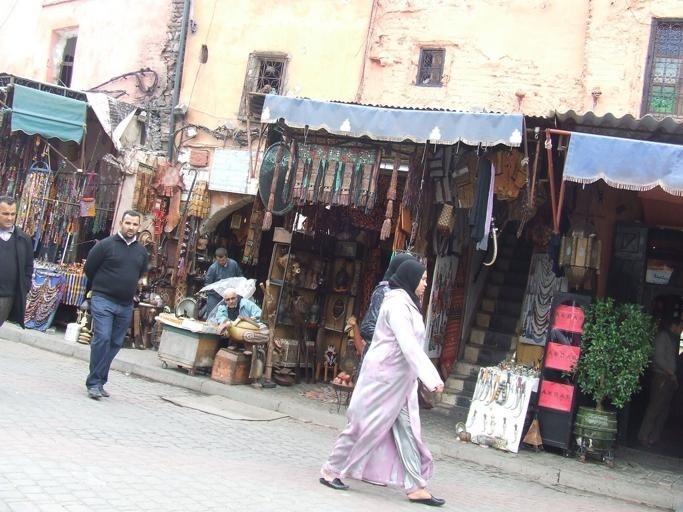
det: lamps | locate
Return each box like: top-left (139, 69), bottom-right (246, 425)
top-left (554, 221), bottom-right (601, 292)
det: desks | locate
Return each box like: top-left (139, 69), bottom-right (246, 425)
top-left (157, 318), bottom-right (220, 379)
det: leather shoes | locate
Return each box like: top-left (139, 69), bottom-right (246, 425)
top-left (409, 494), bottom-right (444, 506)
top-left (319, 477), bottom-right (348, 489)
top-left (87, 385), bottom-right (110, 397)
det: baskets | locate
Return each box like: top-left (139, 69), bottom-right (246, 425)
top-left (437, 204), bottom-right (456, 236)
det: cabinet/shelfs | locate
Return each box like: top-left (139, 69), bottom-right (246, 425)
top-left (255, 226), bottom-right (353, 387)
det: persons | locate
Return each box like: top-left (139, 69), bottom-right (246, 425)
top-left (79, 209), bottom-right (151, 399)
top-left (636, 312), bottom-right (682, 447)
top-left (315, 256), bottom-right (448, 508)
top-left (205, 245), bottom-right (244, 321)
top-left (213, 288), bottom-right (263, 326)
top-left (0, 192), bottom-right (33, 332)
top-left (355, 254), bottom-right (422, 486)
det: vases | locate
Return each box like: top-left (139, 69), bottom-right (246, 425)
top-left (323, 344), bottom-right (337, 367)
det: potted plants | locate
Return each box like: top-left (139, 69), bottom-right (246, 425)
top-left (557, 293), bottom-right (654, 464)
